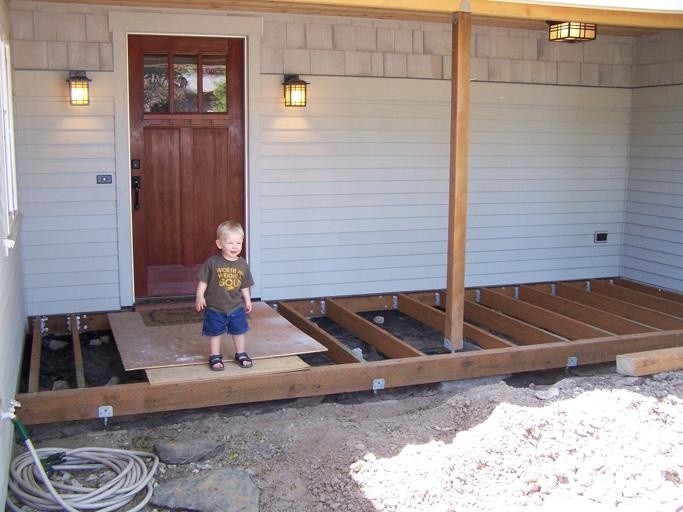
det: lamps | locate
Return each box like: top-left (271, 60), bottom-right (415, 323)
top-left (66, 70), bottom-right (92, 106)
top-left (282, 73), bottom-right (310, 107)
top-left (549, 21), bottom-right (596, 42)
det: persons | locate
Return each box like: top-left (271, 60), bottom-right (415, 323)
top-left (194, 221), bottom-right (254, 371)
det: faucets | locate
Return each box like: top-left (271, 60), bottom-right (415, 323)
top-left (1, 396), bottom-right (22, 424)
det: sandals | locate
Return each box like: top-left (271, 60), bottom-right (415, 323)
top-left (209, 354), bottom-right (224, 370)
top-left (234, 353), bottom-right (252, 368)
